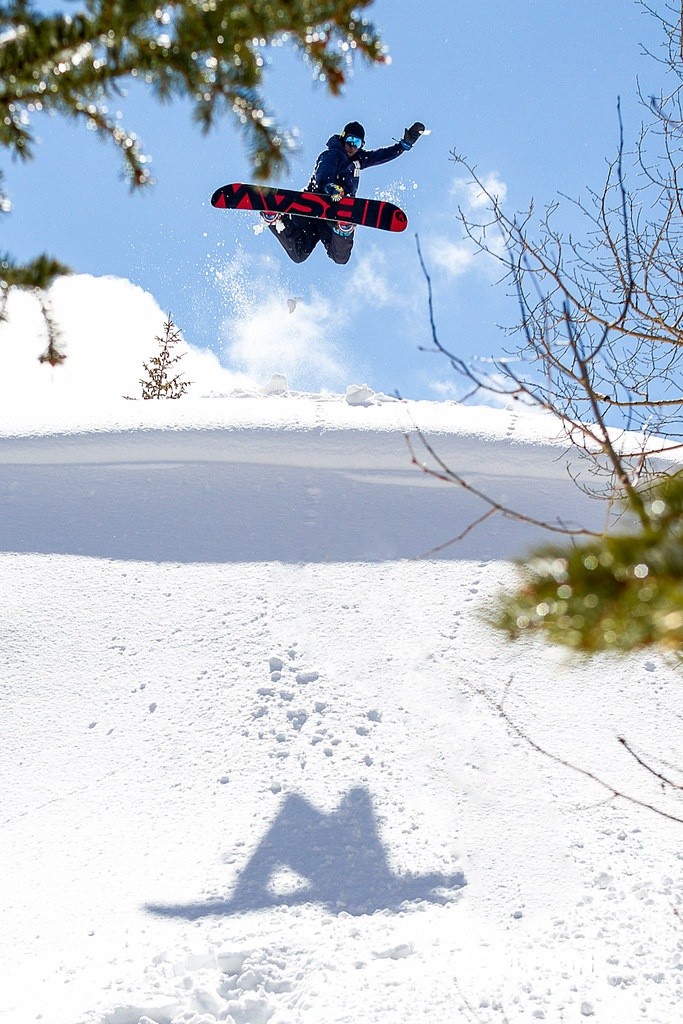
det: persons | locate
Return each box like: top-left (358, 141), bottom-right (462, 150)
top-left (260, 121), bottom-right (425, 265)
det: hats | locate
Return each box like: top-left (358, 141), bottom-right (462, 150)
top-left (339, 121), bottom-right (365, 141)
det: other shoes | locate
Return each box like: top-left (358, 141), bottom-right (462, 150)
top-left (260, 211), bottom-right (282, 225)
top-left (335, 221), bottom-right (356, 236)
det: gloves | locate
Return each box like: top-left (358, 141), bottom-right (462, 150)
top-left (324, 182), bottom-right (345, 202)
top-left (400, 121), bottom-right (425, 151)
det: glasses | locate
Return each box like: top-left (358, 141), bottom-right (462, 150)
top-left (345, 135), bottom-right (364, 149)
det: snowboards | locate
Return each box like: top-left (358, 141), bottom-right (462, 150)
top-left (206, 181), bottom-right (411, 233)
